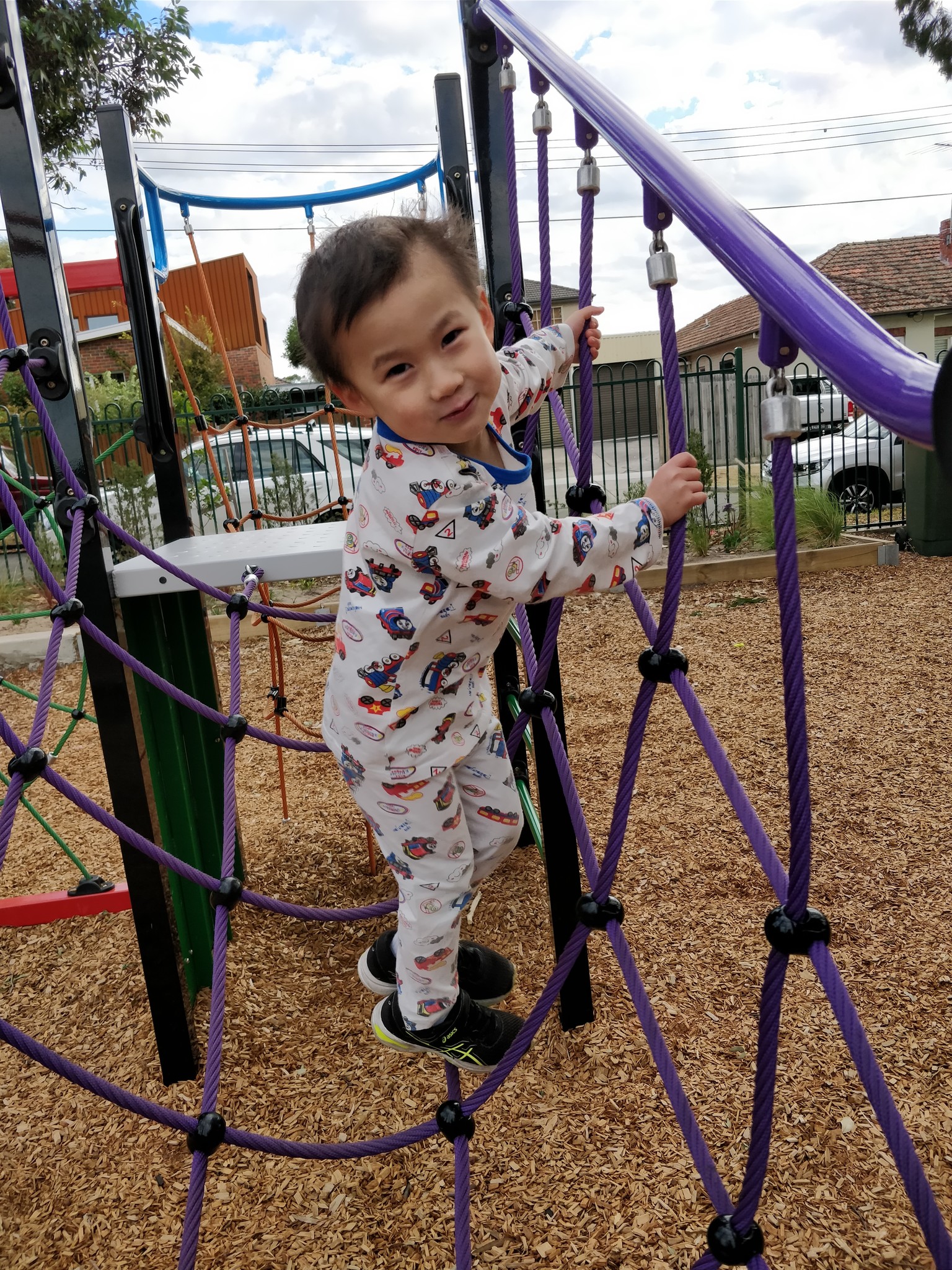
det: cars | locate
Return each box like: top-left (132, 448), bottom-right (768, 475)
top-left (34, 424), bottom-right (374, 573)
top-left (0, 445), bottom-right (56, 531)
top-left (761, 415), bottom-right (905, 517)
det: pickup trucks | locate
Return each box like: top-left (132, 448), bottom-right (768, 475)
top-left (783, 375), bottom-right (865, 437)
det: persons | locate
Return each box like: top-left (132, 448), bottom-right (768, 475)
top-left (295, 199), bottom-right (708, 1075)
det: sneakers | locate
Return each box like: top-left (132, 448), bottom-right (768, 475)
top-left (371, 989), bottom-right (531, 1073)
top-left (357, 929), bottom-right (515, 1007)
